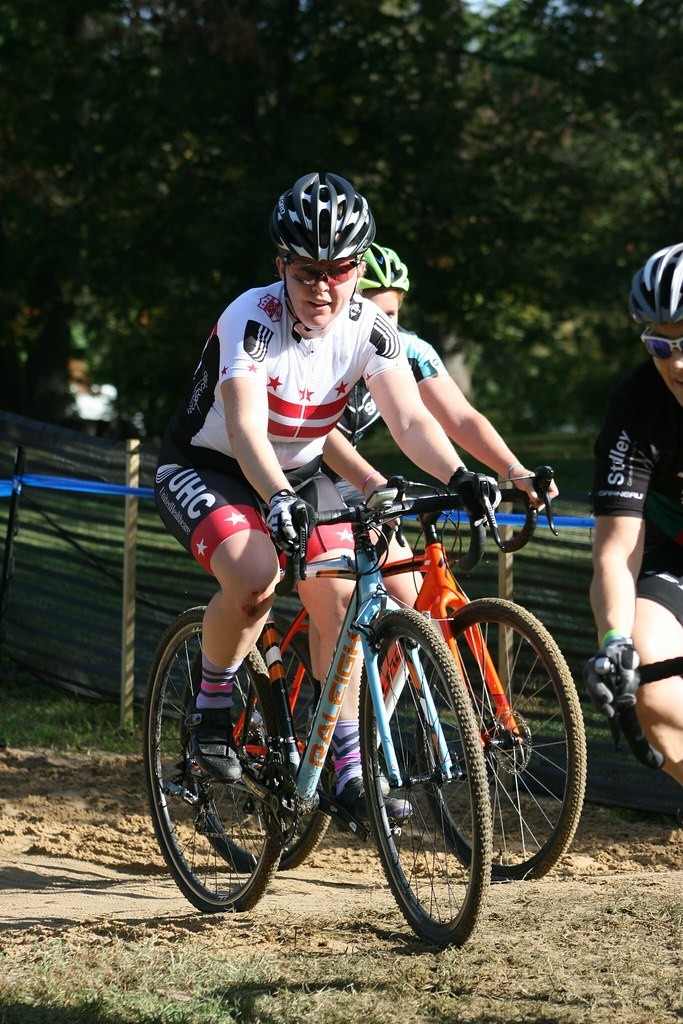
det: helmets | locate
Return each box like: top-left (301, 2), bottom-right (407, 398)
top-left (268, 173), bottom-right (377, 264)
top-left (628, 242), bottom-right (682, 324)
top-left (356, 242), bottom-right (411, 295)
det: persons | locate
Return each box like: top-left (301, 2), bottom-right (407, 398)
top-left (584, 242), bottom-right (682, 782)
top-left (310, 241), bottom-right (559, 796)
top-left (154, 169), bottom-right (502, 833)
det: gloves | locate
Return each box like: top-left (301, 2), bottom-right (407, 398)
top-left (266, 489), bottom-right (317, 554)
top-left (584, 637), bottom-right (644, 719)
top-left (446, 467), bottom-right (502, 518)
top-left (381, 511), bottom-right (403, 531)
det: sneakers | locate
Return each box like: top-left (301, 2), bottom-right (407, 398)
top-left (182, 704), bottom-right (244, 784)
top-left (326, 776), bottom-right (414, 833)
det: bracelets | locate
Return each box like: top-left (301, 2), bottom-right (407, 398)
top-left (596, 628), bottom-right (631, 649)
top-left (361, 471), bottom-right (381, 495)
top-left (508, 464), bottom-right (525, 485)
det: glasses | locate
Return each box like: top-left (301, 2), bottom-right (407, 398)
top-left (283, 256), bottom-right (364, 283)
top-left (641, 326), bottom-right (683, 358)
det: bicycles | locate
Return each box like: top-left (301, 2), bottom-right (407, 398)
top-left (139, 466), bottom-right (495, 948)
top-left (588, 655), bottom-right (683, 769)
top-left (180, 466), bottom-right (587, 884)
